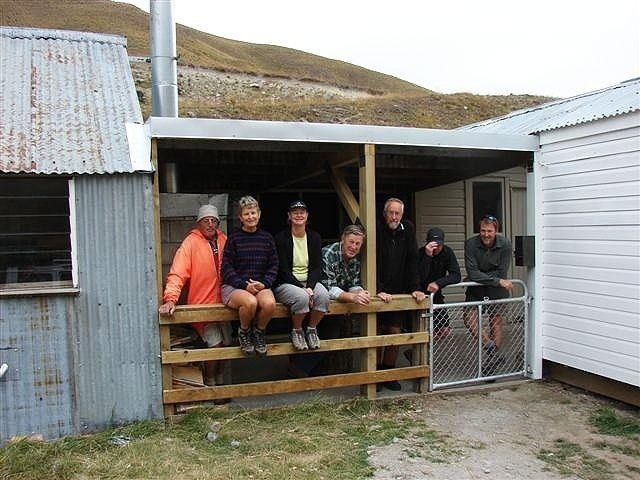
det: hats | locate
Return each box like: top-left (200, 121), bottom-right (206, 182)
top-left (425, 227), bottom-right (448, 247)
top-left (286, 199), bottom-right (305, 211)
top-left (196, 204), bottom-right (221, 226)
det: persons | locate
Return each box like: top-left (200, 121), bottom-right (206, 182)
top-left (403, 227), bottom-right (461, 368)
top-left (220, 195), bottom-right (279, 358)
top-left (320, 225), bottom-right (372, 305)
top-left (159, 204), bottom-right (233, 404)
top-left (375, 198), bottom-right (426, 393)
top-left (275, 198), bottom-right (330, 352)
top-left (462, 215), bottom-right (513, 382)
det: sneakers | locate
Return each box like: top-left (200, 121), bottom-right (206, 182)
top-left (252, 329), bottom-right (268, 355)
top-left (384, 369), bottom-right (403, 390)
top-left (483, 351), bottom-right (504, 373)
top-left (291, 328), bottom-right (308, 351)
top-left (386, 377), bottom-right (400, 391)
top-left (375, 382), bottom-right (385, 391)
top-left (239, 328), bottom-right (255, 355)
top-left (376, 376), bottom-right (383, 391)
top-left (305, 328), bottom-right (321, 350)
top-left (489, 375), bottom-right (497, 382)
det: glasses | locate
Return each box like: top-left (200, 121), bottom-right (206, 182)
top-left (199, 218), bottom-right (218, 223)
top-left (479, 216), bottom-right (496, 222)
